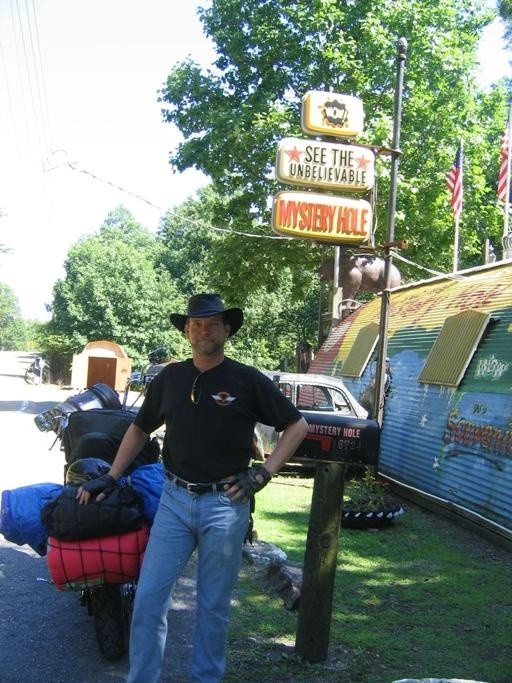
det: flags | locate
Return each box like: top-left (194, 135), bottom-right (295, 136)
top-left (447, 138), bottom-right (462, 221)
top-left (495, 112), bottom-right (510, 204)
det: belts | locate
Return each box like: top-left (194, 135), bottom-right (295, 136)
top-left (164, 471), bottom-right (240, 497)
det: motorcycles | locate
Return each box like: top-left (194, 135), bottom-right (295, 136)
top-left (36, 347), bottom-right (171, 662)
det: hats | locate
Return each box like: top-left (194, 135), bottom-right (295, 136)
top-left (169, 293), bottom-right (244, 337)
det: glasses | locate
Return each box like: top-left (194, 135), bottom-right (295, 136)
top-left (190, 373), bottom-right (203, 405)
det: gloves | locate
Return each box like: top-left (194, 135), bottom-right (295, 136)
top-left (80, 473), bottom-right (117, 497)
top-left (235, 465), bottom-right (272, 500)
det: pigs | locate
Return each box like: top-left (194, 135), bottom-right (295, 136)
top-left (314, 253), bottom-right (402, 307)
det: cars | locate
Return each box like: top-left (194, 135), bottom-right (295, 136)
top-left (254, 370), bottom-right (370, 468)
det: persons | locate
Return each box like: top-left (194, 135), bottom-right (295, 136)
top-left (76, 294), bottom-right (308, 683)
